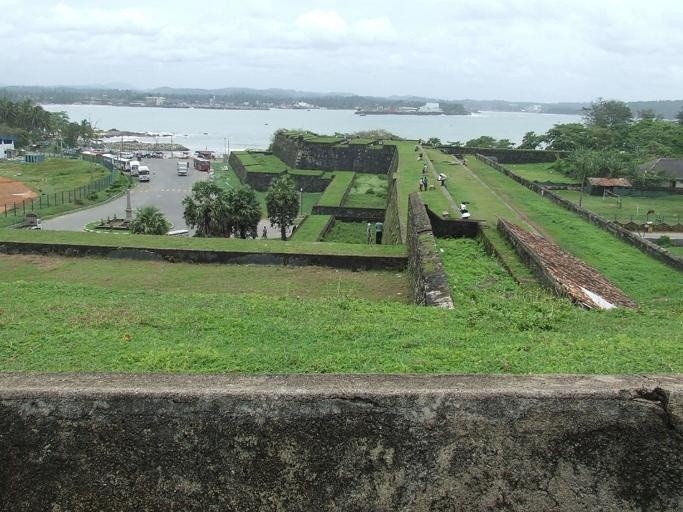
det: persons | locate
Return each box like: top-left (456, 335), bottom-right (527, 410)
top-left (367, 220), bottom-right (383, 244)
top-left (459, 199), bottom-right (471, 220)
top-left (262, 226), bottom-right (267, 238)
top-left (463, 161), bottom-right (467, 166)
top-left (439, 174), bottom-right (445, 185)
top-left (419, 176), bottom-right (428, 191)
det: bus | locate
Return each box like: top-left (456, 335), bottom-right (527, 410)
top-left (81, 148), bottom-right (150, 181)
top-left (193, 158), bottom-right (210, 170)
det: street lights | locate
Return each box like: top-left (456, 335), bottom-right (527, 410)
top-left (162, 134), bottom-right (173, 158)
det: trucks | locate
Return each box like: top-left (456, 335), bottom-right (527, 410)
top-left (6, 213), bottom-right (42, 229)
top-left (176, 161), bottom-right (188, 176)
